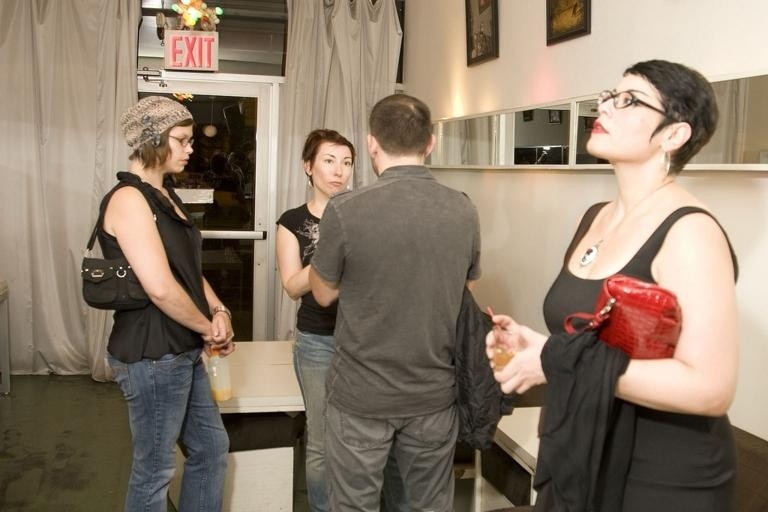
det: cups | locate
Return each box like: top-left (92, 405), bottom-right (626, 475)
top-left (493, 323), bottom-right (519, 372)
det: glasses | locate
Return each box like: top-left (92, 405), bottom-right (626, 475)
top-left (597, 91), bottom-right (666, 116)
top-left (169, 136), bottom-right (195, 147)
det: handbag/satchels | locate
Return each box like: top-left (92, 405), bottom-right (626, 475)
top-left (564, 274), bottom-right (682, 359)
top-left (81, 182), bottom-right (157, 310)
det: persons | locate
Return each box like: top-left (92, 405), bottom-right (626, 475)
top-left (96, 96), bottom-right (235, 512)
top-left (485, 60), bottom-right (740, 511)
top-left (156, 142), bottom-right (255, 297)
top-left (310, 94), bottom-right (480, 511)
top-left (276, 130), bottom-right (412, 511)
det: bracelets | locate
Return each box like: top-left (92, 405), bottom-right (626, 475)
top-left (211, 305), bottom-right (231, 319)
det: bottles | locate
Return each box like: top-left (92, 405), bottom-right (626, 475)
top-left (208, 345), bottom-right (233, 403)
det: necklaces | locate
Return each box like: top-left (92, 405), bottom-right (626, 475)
top-left (580, 180), bottom-right (675, 266)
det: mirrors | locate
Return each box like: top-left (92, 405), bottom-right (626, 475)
top-left (500, 99), bottom-right (572, 167)
top-left (436, 110), bottom-right (501, 167)
top-left (574, 71), bottom-right (768, 173)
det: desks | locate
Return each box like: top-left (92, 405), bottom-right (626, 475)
top-left (200, 341), bottom-right (310, 509)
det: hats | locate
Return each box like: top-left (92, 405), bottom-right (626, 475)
top-left (119, 96), bottom-right (193, 158)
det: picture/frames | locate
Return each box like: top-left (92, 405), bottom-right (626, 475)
top-left (522, 110), bottom-right (534, 122)
top-left (583, 117), bottom-right (595, 131)
top-left (545, 0), bottom-right (590, 47)
top-left (466, 0), bottom-right (498, 64)
top-left (547, 108), bottom-right (562, 124)
